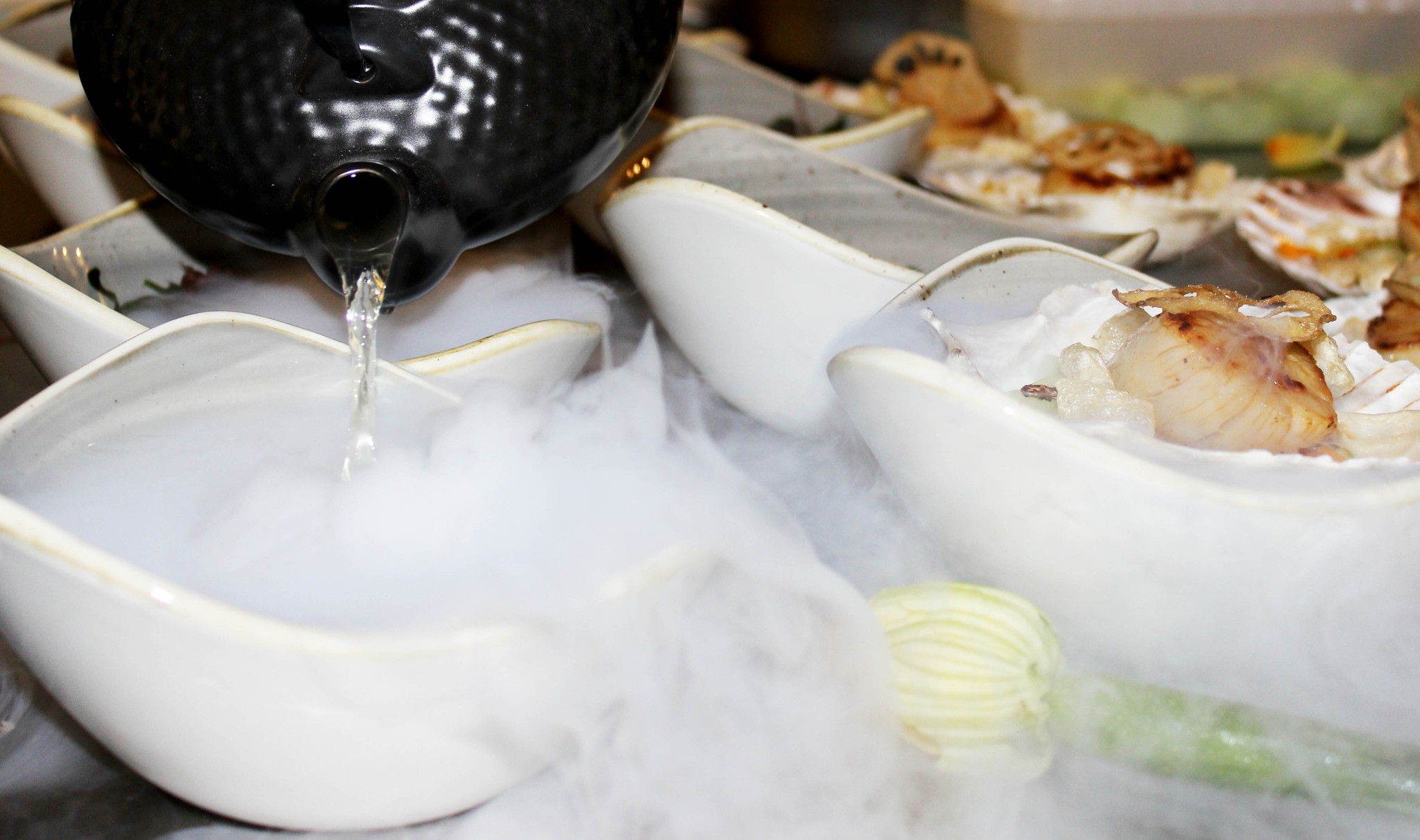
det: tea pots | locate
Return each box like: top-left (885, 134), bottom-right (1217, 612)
top-left (72, 0), bottom-right (683, 307)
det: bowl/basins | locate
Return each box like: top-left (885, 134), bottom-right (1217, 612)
top-left (916, 137), bottom-right (1243, 266)
top-left (0, 8), bottom-right (606, 418)
top-left (1, 316), bottom-right (736, 835)
top-left (823, 237), bottom-right (1419, 783)
top-left (669, 28), bottom-right (1071, 177)
top-left (600, 115), bottom-right (1157, 446)
